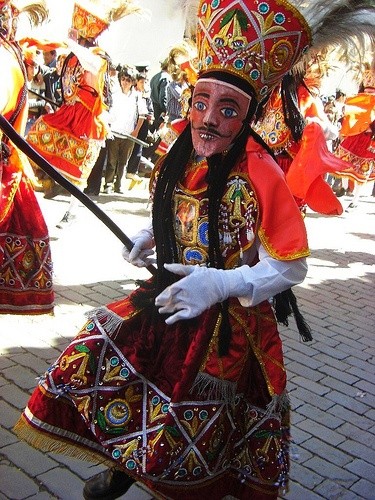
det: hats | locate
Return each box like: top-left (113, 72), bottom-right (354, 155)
top-left (362, 58), bottom-right (375, 88)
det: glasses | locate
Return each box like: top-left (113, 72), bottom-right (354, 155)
top-left (120, 77), bottom-right (132, 83)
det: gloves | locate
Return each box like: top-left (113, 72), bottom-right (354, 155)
top-left (154, 262), bottom-right (250, 326)
top-left (123, 220), bottom-right (158, 270)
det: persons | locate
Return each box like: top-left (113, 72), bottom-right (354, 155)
top-left (15, 0), bottom-right (375, 500)
top-left (0, 0), bottom-right (375, 316)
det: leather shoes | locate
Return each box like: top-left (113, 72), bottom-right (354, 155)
top-left (82, 467), bottom-right (136, 500)
